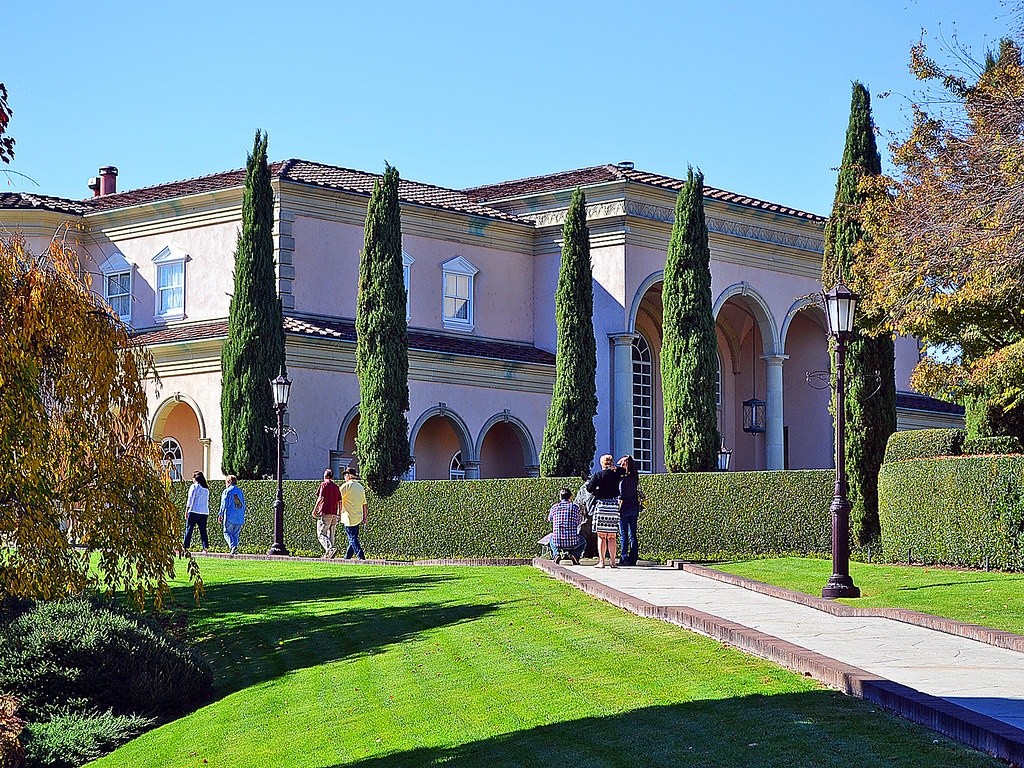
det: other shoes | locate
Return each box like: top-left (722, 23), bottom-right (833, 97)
top-left (617, 559), bottom-right (636, 566)
top-left (571, 553), bottom-right (581, 565)
top-left (610, 563), bottom-right (618, 568)
top-left (594, 563), bottom-right (605, 568)
top-left (553, 554), bottom-right (562, 564)
top-left (231, 546), bottom-right (239, 554)
top-left (329, 549), bottom-right (338, 559)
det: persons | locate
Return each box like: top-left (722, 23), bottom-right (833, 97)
top-left (548, 488), bottom-right (587, 565)
top-left (312, 469), bottom-right (343, 558)
top-left (616, 455), bottom-right (639, 566)
top-left (218, 475), bottom-right (244, 554)
top-left (587, 454), bottom-right (626, 568)
top-left (183, 470), bottom-right (209, 551)
top-left (339, 468), bottom-right (368, 560)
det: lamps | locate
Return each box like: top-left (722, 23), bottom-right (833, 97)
top-left (741, 322), bottom-right (765, 435)
top-left (717, 438), bottom-right (733, 471)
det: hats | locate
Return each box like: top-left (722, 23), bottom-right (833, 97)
top-left (342, 467), bottom-right (360, 477)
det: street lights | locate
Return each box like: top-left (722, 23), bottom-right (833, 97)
top-left (265, 364), bottom-right (294, 557)
top-left (815, 275), bottom-right (862, 598)
